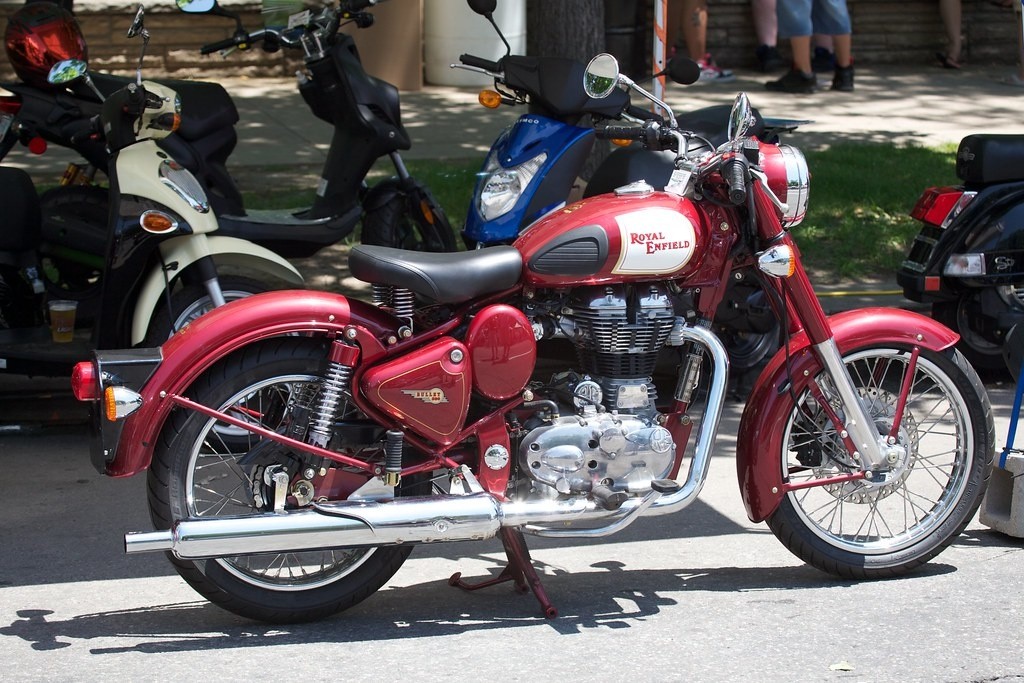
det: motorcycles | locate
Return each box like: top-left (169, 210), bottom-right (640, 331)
top-left (77, 53), bottom-right (996, 623)
top-left (897, 134), bottom-right (1023, 382)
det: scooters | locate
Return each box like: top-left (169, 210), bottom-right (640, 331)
top-left (450, 2), bottom-right (813, 374)
top-left (0, 5), bottom-right (306, 452)
top-left (0, 1), bottom-right (463, 259)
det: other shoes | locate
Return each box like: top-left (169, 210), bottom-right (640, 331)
top-left (748, 42), bottom-right (781, 70)
top-left (811, 45), bottom-right (837, 69)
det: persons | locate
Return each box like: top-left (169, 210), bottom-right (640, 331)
top-left (750, 0), bottom-right (836, 73)
top-left (933, 0), bottom-right (1024, 88)
top-left (665, 0), bottom-right (736, 83)
top-left (765, 0), bottom-right (854, 91)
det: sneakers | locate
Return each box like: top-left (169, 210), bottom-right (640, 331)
top-left (644, 48), bottom-right (736, 82)
top-left (829, 62), bottom-right (853, 93)
top-left (763, 66), bottom-right (815, 90)
